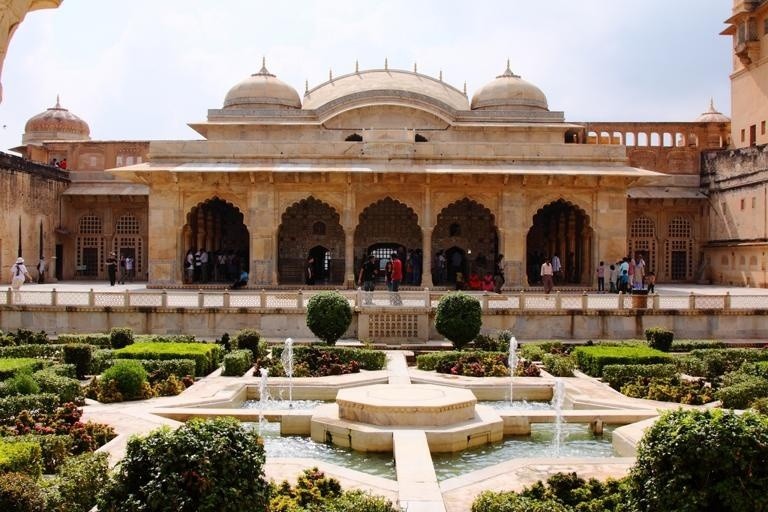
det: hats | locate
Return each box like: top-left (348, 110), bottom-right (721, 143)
top-left (16, 257), bottom-right (24, 264)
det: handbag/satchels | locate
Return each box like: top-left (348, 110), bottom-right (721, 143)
top-left (184, 260), bottom-right (190, 268)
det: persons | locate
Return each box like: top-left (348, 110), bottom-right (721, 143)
top-left (8, 257), bottom-right (33, 302)
top-left (384, 262), bottom-right (392, 289)
top-left (105, 252), bottom-right (118, 286)
top-left (403, 246), bottom-right (656, 294)
top-left (390, 252), bottom-right (401, 305)
top-left (122, 255), bottom-right (135, 284)
top-left (184, 249), bottom-right (248, 285)
top-left (49, 158), bottom-right (57, 168)
top-left (304, 256), bottom-right (316, 286)
top-left (56, 162), bottom-right (60, 168)
top-left (36, 256), bottom-right (48, 284)
top-left (228, 267), bottom-right (249, 288)
top-left (358, 254), bottom-right (379, 305)
top-left (60, 158), bottom-right (66, 169)
top-left (118, 255), bottom-right (125, 284)
